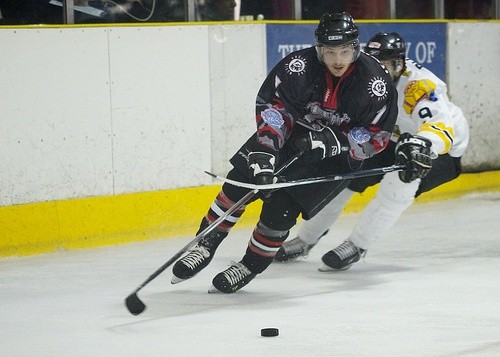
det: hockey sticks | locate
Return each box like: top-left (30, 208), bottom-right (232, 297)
top-left (204, 163), bottom-right (407, 189)
top-left (125, 148), bottom-right (306, 316)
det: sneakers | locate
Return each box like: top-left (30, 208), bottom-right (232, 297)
top-left (171, 240), bottom-right (215, 285)
top-left (317, 237), bottom-right (368, 273)
top-left (208, 261), bottom-right (256, 295)
top-left (275, 236), bottom-right (308, 263)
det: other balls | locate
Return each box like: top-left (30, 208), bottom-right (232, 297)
top-left (261, 328), bottom-right (280, 336)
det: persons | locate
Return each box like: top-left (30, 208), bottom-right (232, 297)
top-left (272, 32), bottom-right (470, 272)
top-left (170, 11), bottom-right (399, 295)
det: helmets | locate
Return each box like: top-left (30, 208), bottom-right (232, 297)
top-left (364, 32), bottom-right (406, 78)
top-left (314, 11), bottom-right (359, 63)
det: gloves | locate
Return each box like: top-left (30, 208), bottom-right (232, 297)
top-left (293, 125), bottom-right (348, 161)
top-left (247, 145), bottom-right (284, 192)
top-left (394, 132), bottom-right (433, 184)
